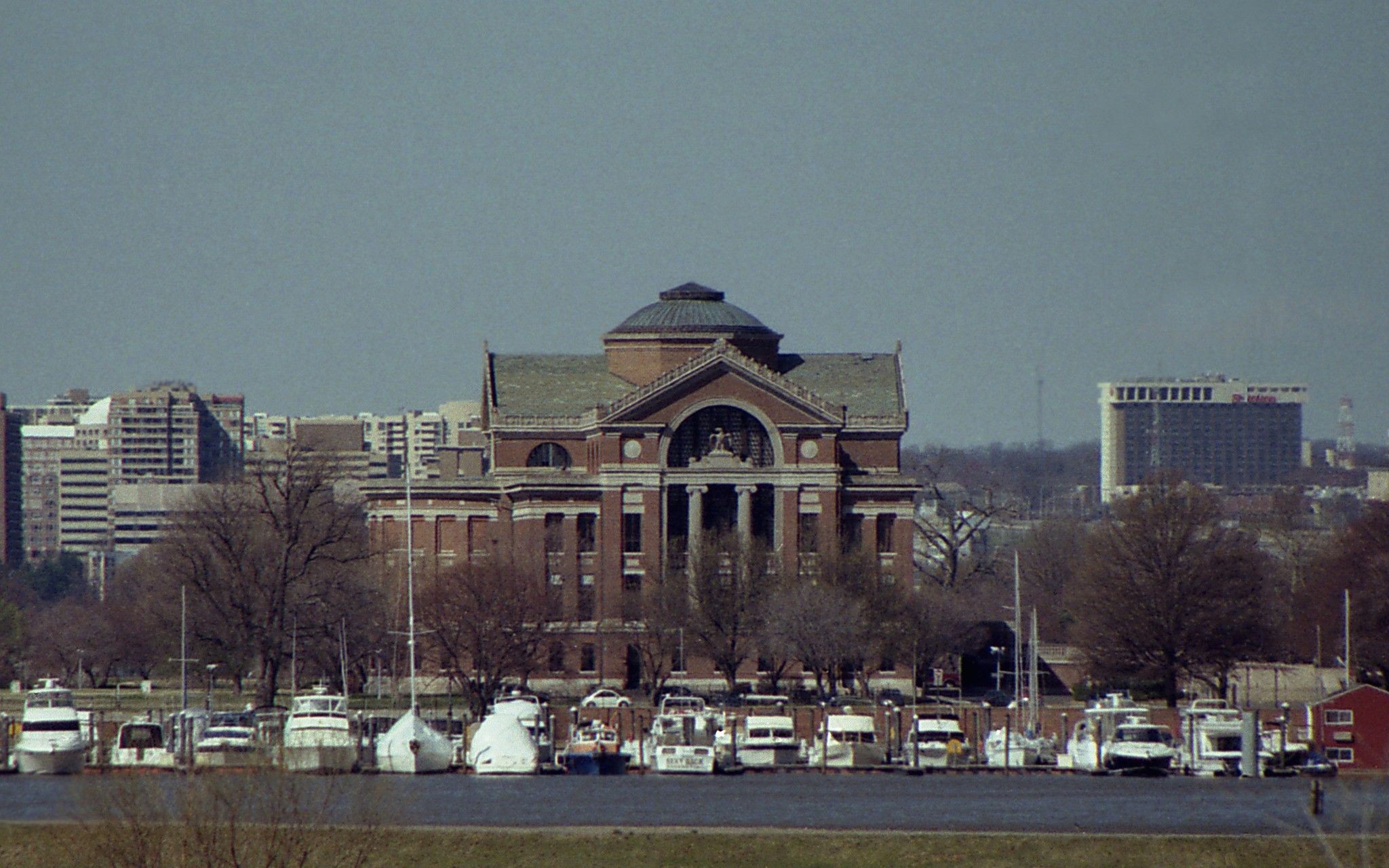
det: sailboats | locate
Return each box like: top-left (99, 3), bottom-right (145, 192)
top-left (377, 460), bottom-right (453, 776)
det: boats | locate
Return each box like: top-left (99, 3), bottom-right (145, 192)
top-left (555, 719), bottom-right (633, 776)
top-left (189, 709), bottom-right (269, 767)
top-left (109, 711), bottom-right (175, 770)
top-left (8, 671), bottom-right (87, 775)
top-left (643, 692), bottom-right (1340, 780)
top-left (283, 678), bottom-right (355, 773)
top-left (462, 688), bottom-right (556, 774)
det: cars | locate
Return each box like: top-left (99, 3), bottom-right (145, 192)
top-left (654, 682), bottom-right (908, 708)
top-left (582, 689), bottom-right (631, 708)
